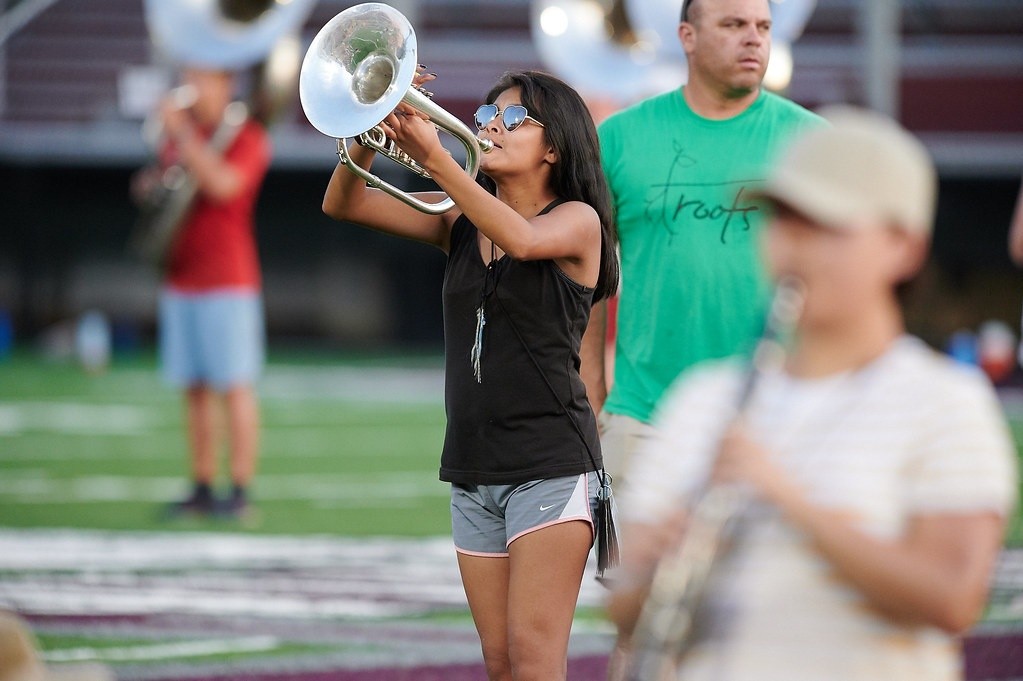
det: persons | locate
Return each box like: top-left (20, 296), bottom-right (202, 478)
top-left (136, 53), bottom-right (272, 522)
top-left (321, 64), bottom-right (620, 681)
top-left (580, 1), bottom-right (1023, 681)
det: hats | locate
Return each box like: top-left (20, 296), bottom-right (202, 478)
top-left (733, 102), bottom-right (939, 237)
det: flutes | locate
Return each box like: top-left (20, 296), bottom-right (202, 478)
top-left (610, 274), bottom-right (810, 681)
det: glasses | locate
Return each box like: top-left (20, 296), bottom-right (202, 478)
top-left (473, 104), bottom-right (547, 133)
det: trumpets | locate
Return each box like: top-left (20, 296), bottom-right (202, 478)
top-left (298, 2), bottom-right (495, 214)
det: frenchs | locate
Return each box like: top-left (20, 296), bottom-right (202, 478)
top-left (128, 0), bottom-right (306, 258)
